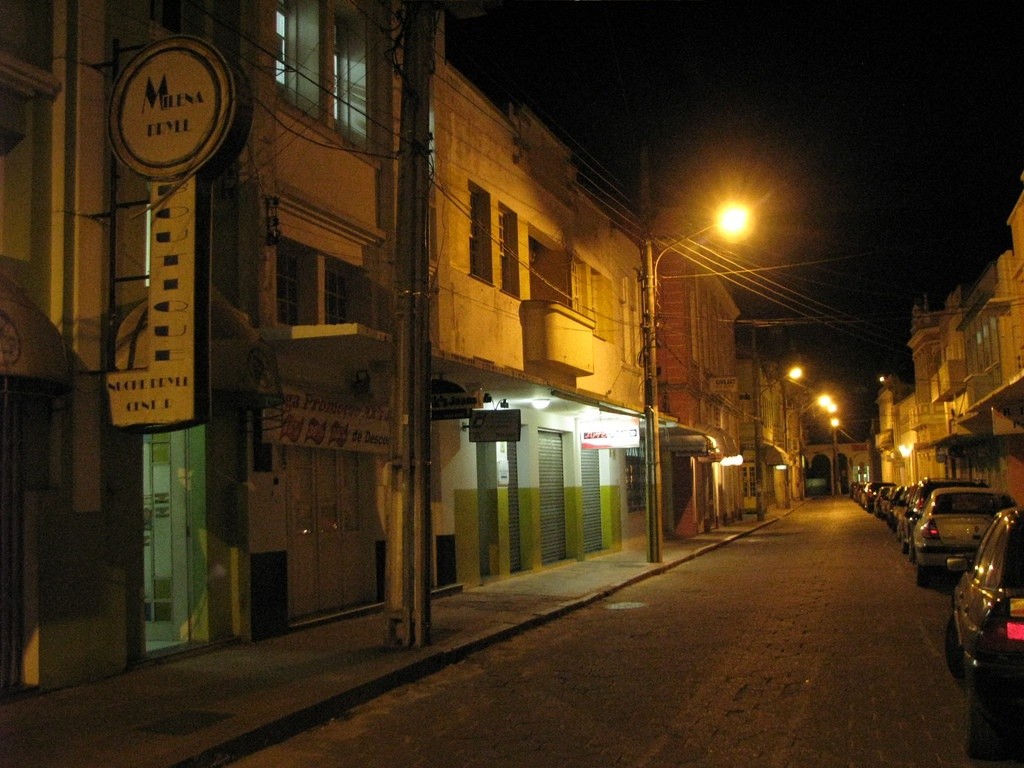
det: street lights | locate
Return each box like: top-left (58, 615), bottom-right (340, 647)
top-left (756, 367), bottom-right (804, 520)
top-left (641, 204), bottom-right (757, 563)
top-left (793, 393), bottom-right (839, 502)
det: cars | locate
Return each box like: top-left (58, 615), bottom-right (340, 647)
top-left (847, 476), bottom-right (992, 565)
top-left (904, 485), bottom-right (1018, 589)
top-left (943, 505), bottom-right (1024, 760)
